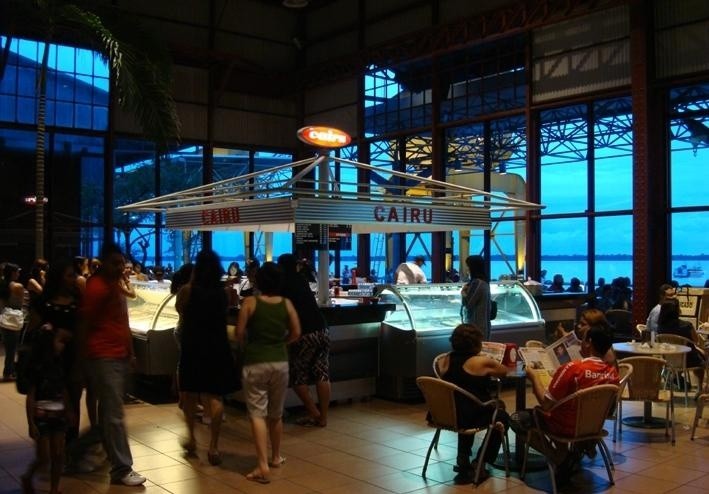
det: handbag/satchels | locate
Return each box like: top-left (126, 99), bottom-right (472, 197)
top-left (1, 308), bottom-right (24, 331)
top-left (488, 301), bottom-right (497, 320)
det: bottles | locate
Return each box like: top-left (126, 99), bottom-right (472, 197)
top-left (334, 286), bottom-right (339, 296)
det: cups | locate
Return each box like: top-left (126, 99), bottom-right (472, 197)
top-left (347, 283), bottom-right (377, 296)
top-left (363, 298), bottom-right (369, 305)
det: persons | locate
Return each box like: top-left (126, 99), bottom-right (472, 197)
top-left (342, 265), bottom-right (349, 285)
top-left (426, 324), bottom-right (507, 484)
top-left (394, 255), bottom-right (428, 284)
top-left (461, 255), bottom-right (491, 342)
top-left (1, 241), bottom-right (343, 493)
top-left (370, 268), bottom-right (378, 283)
top-left (386, 268), bottom-right (395, 283)
top-left (510, 325), bottom-right (623, 485)
top-left (352, 268), bottom-right (373, 283)
top-left (541, 269), bottom-right (709, 416)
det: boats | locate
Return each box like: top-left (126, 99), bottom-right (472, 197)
top-left (672, 263), bottom-right (705, 278)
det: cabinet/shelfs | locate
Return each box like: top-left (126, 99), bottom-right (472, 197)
top-left (125, 278), bottom-right (183, 404)
top-left (373, 283), bottom-right (545, 405)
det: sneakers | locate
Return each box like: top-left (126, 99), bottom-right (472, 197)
top-left (22, 474), bottom-right (39, 493)
top-left (297, 416), bottom-right (312, 426)
top-left (311, 417), bottom-right (328, 426)
top-left (48, 484), bottom-right (66, 494)
top-left (122, 471), bottom-right (146, 486)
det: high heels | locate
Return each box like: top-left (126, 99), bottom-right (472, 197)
top-left (207, 448), bottom-right (223, 465)
top-left (181, 437), bottom-right (197, 453)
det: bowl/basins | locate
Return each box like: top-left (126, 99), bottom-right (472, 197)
top-left (370, 297), bottom-right (381, 304)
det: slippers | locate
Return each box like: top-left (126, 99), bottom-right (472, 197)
top-left (242, 467), bottom-right (270, 484)
top-left (270, 456), bottom-right (287, 468)
